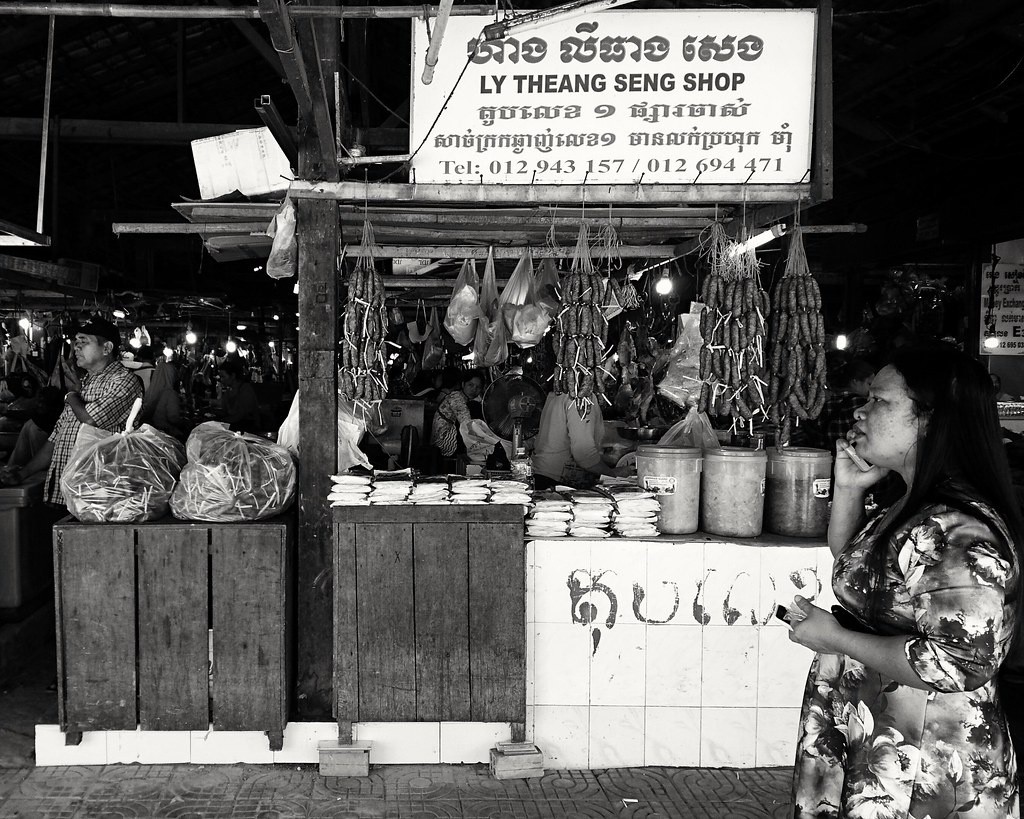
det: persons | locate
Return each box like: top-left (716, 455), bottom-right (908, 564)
top-left (0, 318), bottom-right (497, 511)
top-left (780, 337), bottom-right (1022, 813)
top-left (530, 382), bottom-right (632, 495)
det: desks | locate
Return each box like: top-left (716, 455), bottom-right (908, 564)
top-left (0, 474), bottom-right (53, 609)
top-left (41, 517), bottom-right (290, 751)
top-left (332, 504), bottom-right (530, 745)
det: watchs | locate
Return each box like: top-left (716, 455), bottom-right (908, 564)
top-left (64, 391), bottom-right (77, 404)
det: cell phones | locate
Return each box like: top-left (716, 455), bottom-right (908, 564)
top-left (842, 438), bottom-right (877, 472)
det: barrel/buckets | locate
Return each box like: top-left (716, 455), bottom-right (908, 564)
top-left (702, 445), bottom-right (768, 538)
top-left (634, 443), bottom-right (704, 534)
top-left (764, 446), bottom-right (833, 536)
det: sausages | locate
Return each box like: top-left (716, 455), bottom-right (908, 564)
top-left (342, 269), bottom-right (389, 403)
top-left (60, 432), bottom-right (300, 521)
top-left (552, 274), bottom-right (626, 399)
top-left (698, 275), bottom-right (826, 455)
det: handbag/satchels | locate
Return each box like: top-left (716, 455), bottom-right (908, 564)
top-left (442, 246), bottom-right (561, 366)
top-left (59, 397), bottom-right (186, 524)
top-left (169, 421), bottom-right (296, 523)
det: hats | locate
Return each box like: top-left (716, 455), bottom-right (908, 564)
top-left (68, 316), bottom-right (119, 343)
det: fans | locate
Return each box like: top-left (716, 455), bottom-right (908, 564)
top-left (382, 423), bottom-right (421, 468)
top-left (482, 371), bottom-right (547, 470)
